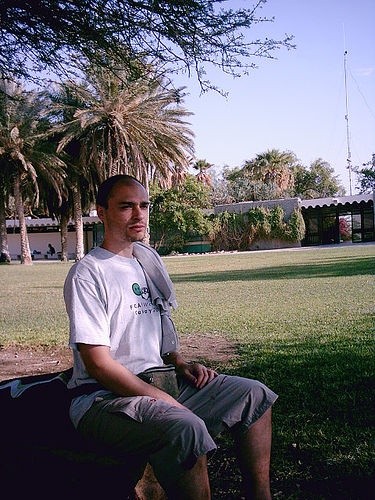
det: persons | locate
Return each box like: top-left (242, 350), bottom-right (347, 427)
top-left (62, 175), bottom-right (280, 500)
top-left (46, 243), bottom-right (56, 256)
